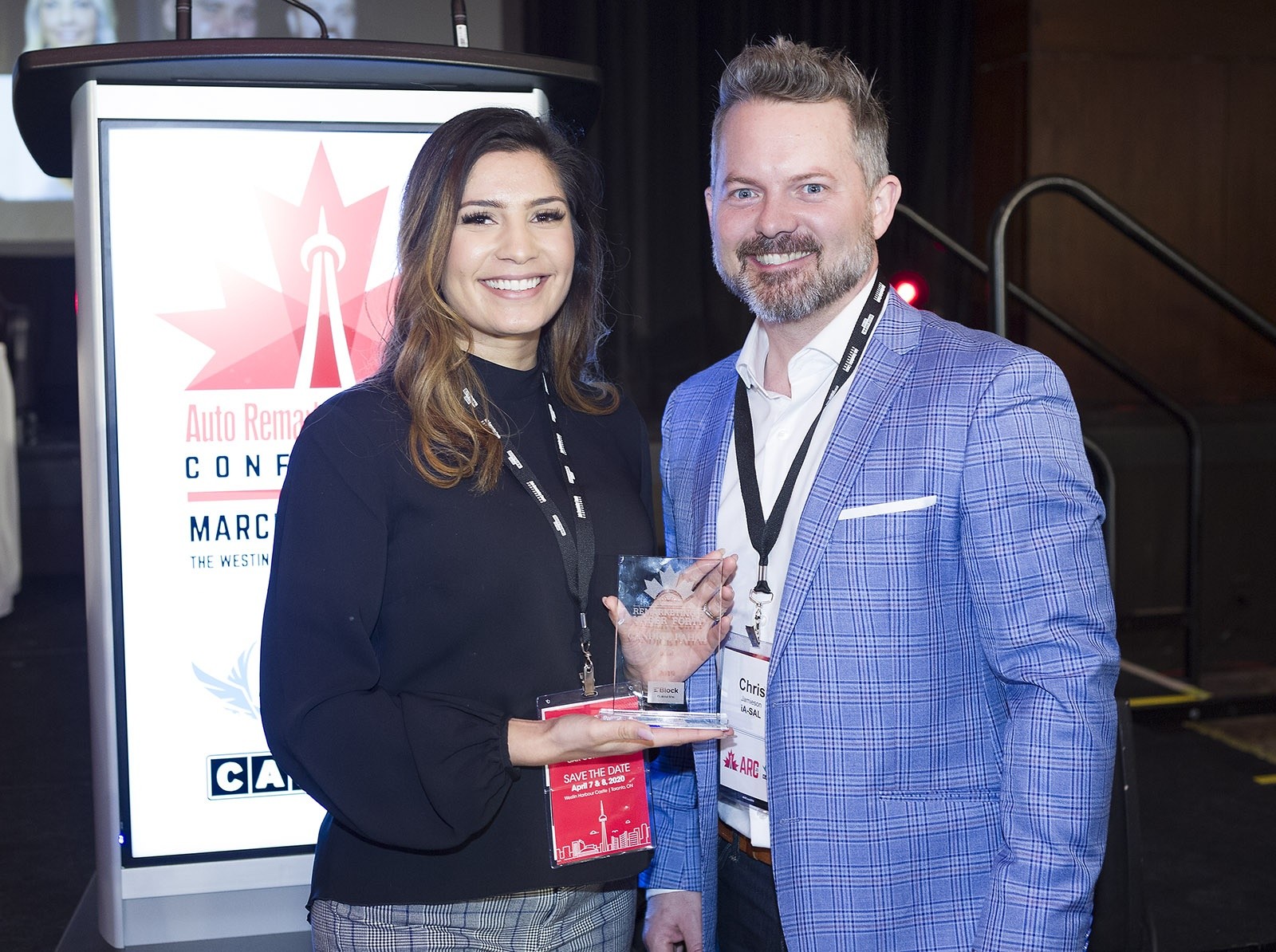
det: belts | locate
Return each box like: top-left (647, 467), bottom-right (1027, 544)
top-left (717, 818), bottom-right (771, 865)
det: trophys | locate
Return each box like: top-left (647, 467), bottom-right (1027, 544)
top-left (600, 556), bottom-right (728, 731)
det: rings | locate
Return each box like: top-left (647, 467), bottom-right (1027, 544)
top-left (703, 602), bottom-right (724, 621)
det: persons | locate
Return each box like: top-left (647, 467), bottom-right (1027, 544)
top-left (259, 108), bottom-right (739, 952)
top-left (640, 44), bottom-right (1120, 952)
